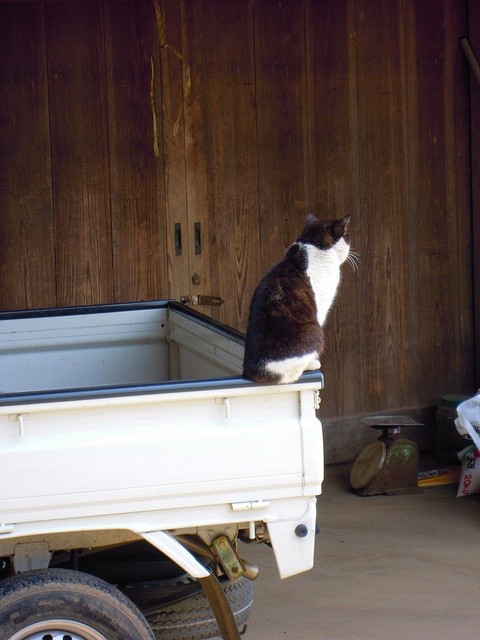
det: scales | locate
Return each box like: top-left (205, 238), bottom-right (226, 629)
top-left (349, 415), bottom-right (425, 497)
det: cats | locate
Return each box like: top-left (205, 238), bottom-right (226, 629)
top-left (242, 211), bottom-right (361, 386)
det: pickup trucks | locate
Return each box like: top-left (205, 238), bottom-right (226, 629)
top-left (0, 300), bottom-right (324, 640)
